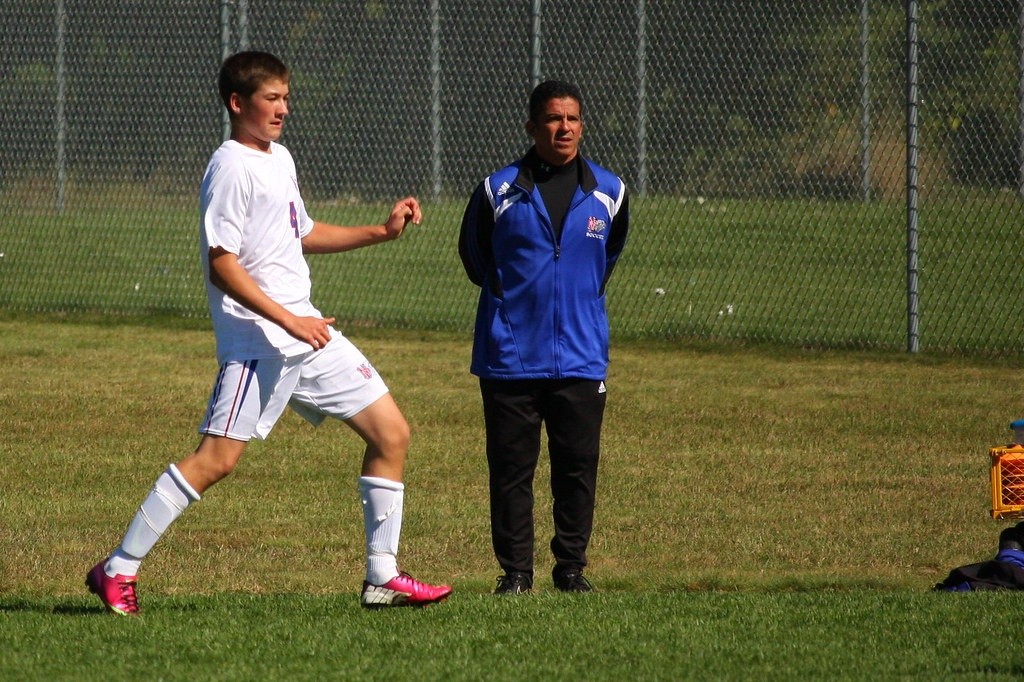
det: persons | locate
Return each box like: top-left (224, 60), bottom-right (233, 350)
top-left (456, 80), bottom-right (638, 596)
top-left (83, 49), bottom-right (451, 616)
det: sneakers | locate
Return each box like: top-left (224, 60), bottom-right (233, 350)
top-left (494, 572), bottom-right (531, 595)
top-left (552, 565), bottom-right (594, 595)
top-left (85, 558), bottom-right (139, 619)
top-left (361, 567), bottom-right (453, 609)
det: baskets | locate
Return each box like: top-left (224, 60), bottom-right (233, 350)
top-left (989, 443), bottom-right (1024, 520)
